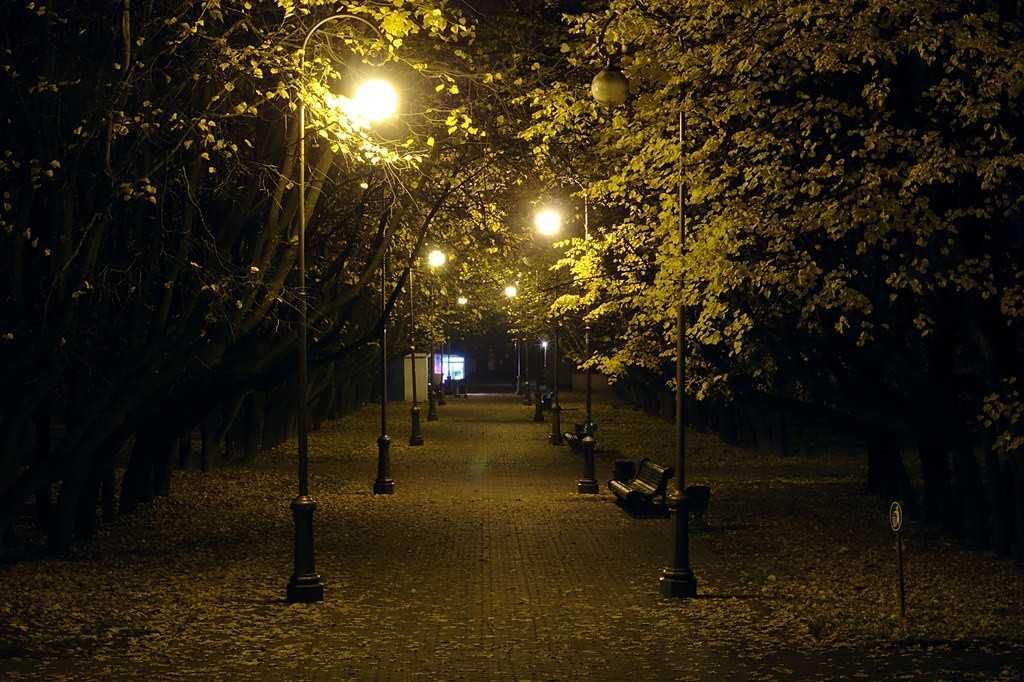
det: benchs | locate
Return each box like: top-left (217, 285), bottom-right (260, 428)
top-left (563, 419), bottom-right (597, 453)
top-left (608, 459), bottom-right (670, 511)
top-left (540, 391), bottom-right (554, 408)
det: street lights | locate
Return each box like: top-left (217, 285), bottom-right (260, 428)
top-left (408, 237), bottom-right (446, 447)
top-left (283, 13), bottom-right (400, 605)
top-left (504, 281), bottom-right (533, 406)
top-left (531, 174), bottom-right (599, 495)
top-left (590, 4), bottom-right (697, 599)
top-left (445, 290), bottom-right (470, 397)
